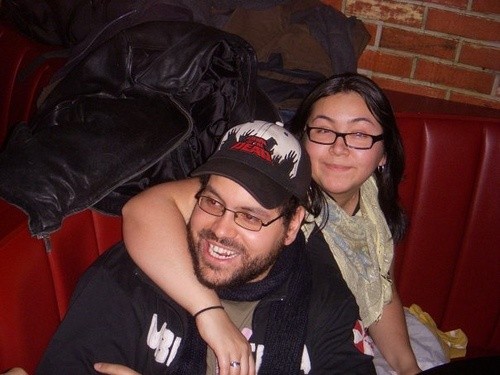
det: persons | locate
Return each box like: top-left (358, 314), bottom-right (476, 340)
top-left (122, 74), bottom-right (425, 375)
top-left (0, 118), bottom-right (377, 375)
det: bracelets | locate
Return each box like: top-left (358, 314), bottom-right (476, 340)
top-left (193, 306), bottom-right (224, 319)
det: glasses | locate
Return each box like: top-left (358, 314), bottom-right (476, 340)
top-left (304, 122), bottom-right (385, 150)
top-left (194, 187), bottom-right (289, 232)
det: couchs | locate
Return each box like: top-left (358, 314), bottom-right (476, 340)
top-left (0, 24), bottom-right (500, 375)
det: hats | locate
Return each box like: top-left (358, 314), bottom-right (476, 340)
top-left (190, 120), bottom-right (311, 209)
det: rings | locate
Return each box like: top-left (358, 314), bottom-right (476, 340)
top-left (230, 361), bottom-right (240, 368)
